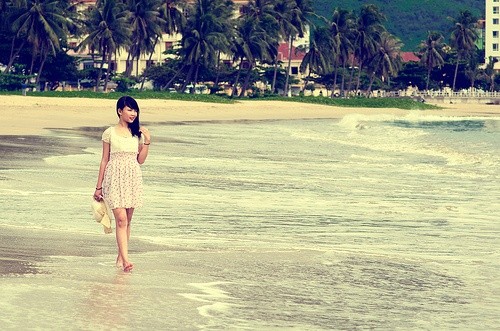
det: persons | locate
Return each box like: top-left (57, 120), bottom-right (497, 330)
top-left (93, 96), bottom-right (151, 272)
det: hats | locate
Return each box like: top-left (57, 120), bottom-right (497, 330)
top-left (90, 195), bottom-right (113, 235)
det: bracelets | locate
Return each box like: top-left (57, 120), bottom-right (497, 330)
top-left (144, 143), bottom-right (150, 145)
top-left (96, 187), bottom-right (102, 190)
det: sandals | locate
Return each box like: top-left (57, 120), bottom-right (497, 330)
top-left (116, 258), bottom-right (134, 273)
